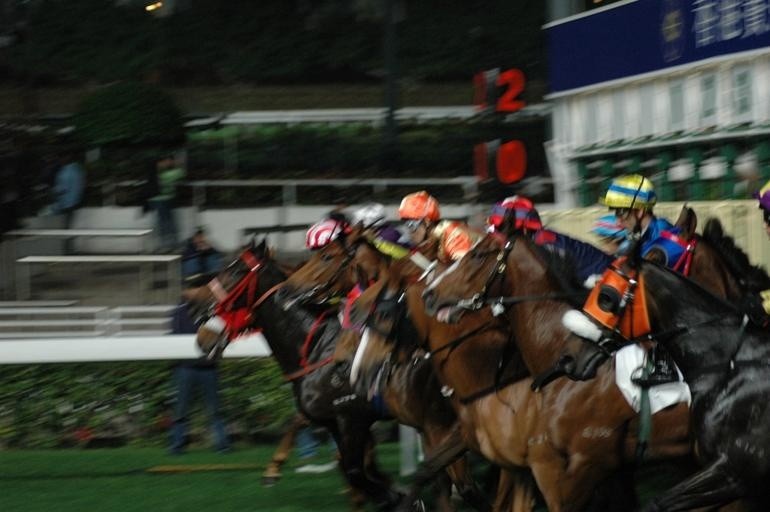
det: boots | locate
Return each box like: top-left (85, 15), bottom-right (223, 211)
top-left (631, 347), bottom-right (679, 385)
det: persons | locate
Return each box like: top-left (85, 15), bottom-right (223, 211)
top-left (303, 218), bottom-right (414, 271)
top-left (397, 188), bottom-right (485, 266)
top-left (487, 195), bottom-right (617, 294)
top-left (603, 173), bottom-right (692, 278)
top-left (744, 178), bottom-right (770, 324)
top-left (162, 278), bottom-right (238, 454)
top-left (48, 146), bottom-right (88, 258)
top-left (136, 148), bottom-right (195, 250)
top-left (181, 226), bottom-right (224, 291)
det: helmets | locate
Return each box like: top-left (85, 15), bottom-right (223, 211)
top-left (400, 192), bottom-right (440, 221)
top-left (354, 202), bottom-right (385, 227)
top-left (605, 174), bottom-right (657, 211)
top-left (488, 195), bottom-right (542, 230)
top-left (307, 220), bottom-right (345, 250)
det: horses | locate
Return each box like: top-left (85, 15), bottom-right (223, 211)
top-left (180, 203), bottom-right (770, 511)
